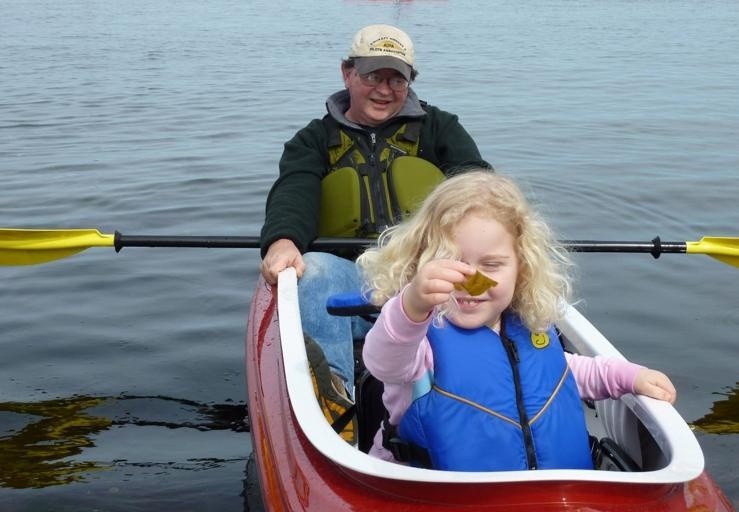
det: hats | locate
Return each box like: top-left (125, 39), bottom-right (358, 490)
top-left (349, 24), bottom-right (414, 82)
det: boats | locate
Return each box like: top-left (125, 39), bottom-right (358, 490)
top-left (241, 230), bottom-right (736, 512)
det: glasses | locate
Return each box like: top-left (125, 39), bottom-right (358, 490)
top-left (354, 72), bottom-right (411, 91)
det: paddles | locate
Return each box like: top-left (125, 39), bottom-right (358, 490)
top-left (551, 237), bottom-right (739, 269)
top-left (0, 228), bottom-right (390, 267)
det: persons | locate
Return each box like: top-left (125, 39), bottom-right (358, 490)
top-left (257, 20), bottom-right (499, 448)
top-left (356, 166), bottom-right (679, 472)
